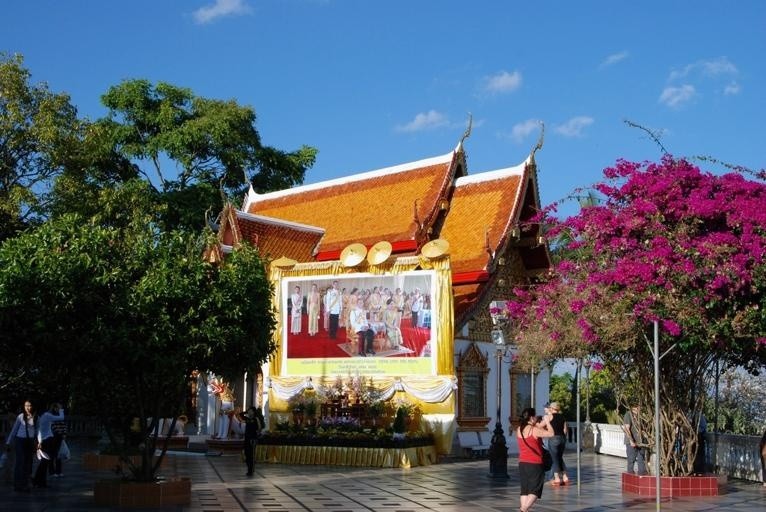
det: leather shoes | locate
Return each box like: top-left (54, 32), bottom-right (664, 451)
top-left (360, 349), bottom-right (375, 356)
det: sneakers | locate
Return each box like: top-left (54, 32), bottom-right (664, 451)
top-left (50, 473), bottom-right (64, 478)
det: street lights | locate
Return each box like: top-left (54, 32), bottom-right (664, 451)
top-left (490, 329), bottom-right (511, 482)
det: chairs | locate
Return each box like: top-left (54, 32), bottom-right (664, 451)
top-left (344, 297), bottom-right (398, 352)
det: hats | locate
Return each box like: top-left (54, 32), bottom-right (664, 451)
top-left (550, 402), bottom-right (561, 410)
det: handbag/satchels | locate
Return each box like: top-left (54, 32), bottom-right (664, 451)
top-left (37, 448), bottom-right (51, 462)
top-left (542, 447), bottom-right (552, 471)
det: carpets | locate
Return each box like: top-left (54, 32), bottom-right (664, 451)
top-left (337, 336), bottom-right (415, 357)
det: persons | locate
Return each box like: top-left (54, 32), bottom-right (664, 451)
top-left (240, 407), bottom-right (263, 476)
top-left (290, 281), bottom-right (430, 358)
top-left (536, 401), bottom-right (569, 486)
top-left (760, 431), bottom-right (766, 472)
top-left (5, 399), bottom-right (70, 493)
top-left (623, 404), bottom-right (708, 473)
top-left (517, 408), bottom-right (555, 512)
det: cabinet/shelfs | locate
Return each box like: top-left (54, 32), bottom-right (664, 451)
top-left (417, 309), bottom-right (431, 328)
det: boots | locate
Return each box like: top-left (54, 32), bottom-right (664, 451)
top-left (246, 464), bottom-right (255, 476)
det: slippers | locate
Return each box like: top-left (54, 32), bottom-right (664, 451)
top-left (548, 479), bottom-right (569, 485)
